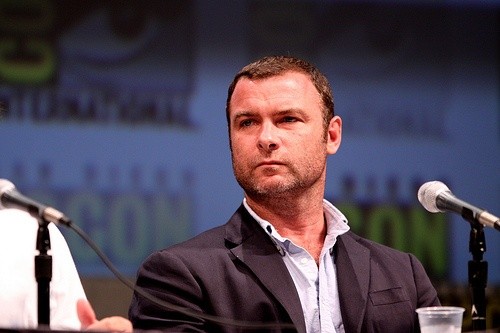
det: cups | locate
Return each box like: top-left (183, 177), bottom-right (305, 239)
top-left (415, 306), bottom-right (466, 333)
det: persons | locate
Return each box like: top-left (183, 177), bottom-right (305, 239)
top-left (0, 208), bottom-right (135, 333)
top-left (124, 53), bottom-right (443, 333)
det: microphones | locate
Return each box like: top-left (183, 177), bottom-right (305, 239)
top-left (0, 177), bottom-right (71, 227)
top-left (418, 180), bottom-right (500, 232)
